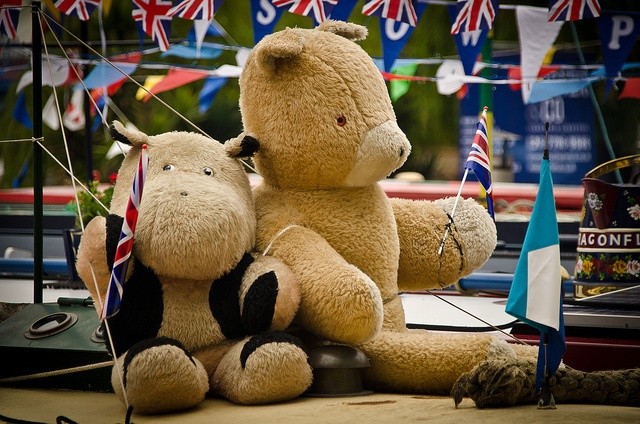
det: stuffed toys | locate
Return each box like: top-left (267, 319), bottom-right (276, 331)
top-left (74, 120), bottom-right (314, 413)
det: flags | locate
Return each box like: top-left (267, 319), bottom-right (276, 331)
top-left (52, 0), bottom-right (102, 21)
top-left (17, 51), bottom-right (69, 91)
top-left (360, 0), bottom-right (418, 28)
top-left (272, 0), bottom-right (340, 25)
top-left (592, 63), bottom-right (640, 75)
top-left (185, 20), bottom-right (222, 59)
top-left (238, 20), bottom-right (566, 392)
top-left (0, 0), bottom-right (21, 40)
top-left (43, 92), bottom-right (59, 131)
top-left (618, 78), bottom-right (640, 98)
top-left (74, 52), bottom-right (142, 116)
top-left (505, 160), bottom-right (565, 386)
top-left (548, 0), bottom-right (602, 23)
top-left (538, 48), bottom-right (557, 80)
top-left (436, 51), bottom-right (482, 99)
top-left (380, 0), bottom-right (428, 72)
top-left (14, 89), bottom-right (32, 130)
top-left (135, 75), bottom-right (166, 101)
top-left (98, 143), bottom-right (149, 321)
top-left (62, 89), bottom-right (86, 132)
top-left (165, 0), bottom-right (224, 20)
top-left (313, 0), bottom-right (358, 26)
top-left (516, 5), bottom-right (566, 105)
top-left (131, 0), bottom-right (173, 53)
top-left (448, 0), bottom-right (499, 75)
top-left (161, 37), bottom-right (223, 59)
top-left (250, 0), bottom-right (283, 46)
top-left (375, 58), bottom-right (418, 101)
top-left (199, 48), bottom-right (253, 113)
top-left (41, 0), bottom-right (64, 39)
top-left (527, 78), bottom-right (595, 104)
top-left (464, 109), bottom-right (495, 222)
top-left (600, 10), bottom-right (640, 96)
top-left (143, 68), bottom-right (208, 103)
top-left (509, 65), bottom-right (561, 90)
